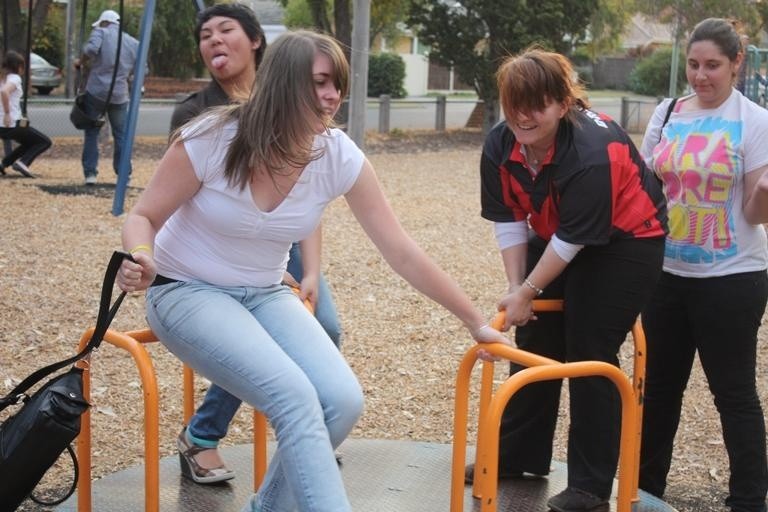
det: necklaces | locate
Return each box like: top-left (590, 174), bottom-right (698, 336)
top-left (529, 145), bottom-right (542, 165)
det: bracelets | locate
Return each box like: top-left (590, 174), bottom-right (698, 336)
top-left (5, 111), bottom-right (10, 114)
top-left (478, 324), bottom-right (489, 335)
top-left (525, 280), bottom-right (544, 296)
top-left (129, 245), bottom-right (151, 254)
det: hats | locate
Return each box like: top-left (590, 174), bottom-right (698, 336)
top-left (91, 10), bottom-right (120, 28)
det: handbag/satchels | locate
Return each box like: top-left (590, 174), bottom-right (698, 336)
top-left (0, 366), bottom-right (90, 512)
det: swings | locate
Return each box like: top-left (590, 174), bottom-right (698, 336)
top-left (0, 1), bottom-right (33, 144)
top-left (70, 1), bottom-right (124, 129)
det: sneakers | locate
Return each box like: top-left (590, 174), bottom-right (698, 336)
top-left (12, 159), bottom-right (35, 178)
top-left (464, 456), bottom-right (522, 483)
top-left (84, 175), bottom-right (96, 185)
top-left (547, 487), bottom-right (609, 512)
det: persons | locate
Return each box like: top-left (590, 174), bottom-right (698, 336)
top-left (638, 17), bottom-right (768, 512)
top-left (117, 30), bottom-right (518, 511)
top-left (0, 50), bottom-right (51, 178)
top-left (167, 3), bottom-right (342, 484)
top-left (72, 10), bottom-right (141, 185)
top-left (465, 44), bottom-right (671, 512)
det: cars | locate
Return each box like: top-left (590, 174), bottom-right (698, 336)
top-left (29, 52), bottom-right (62, 95)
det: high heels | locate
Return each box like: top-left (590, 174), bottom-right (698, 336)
top-left (177, 427), bottom-right (236, 483)
